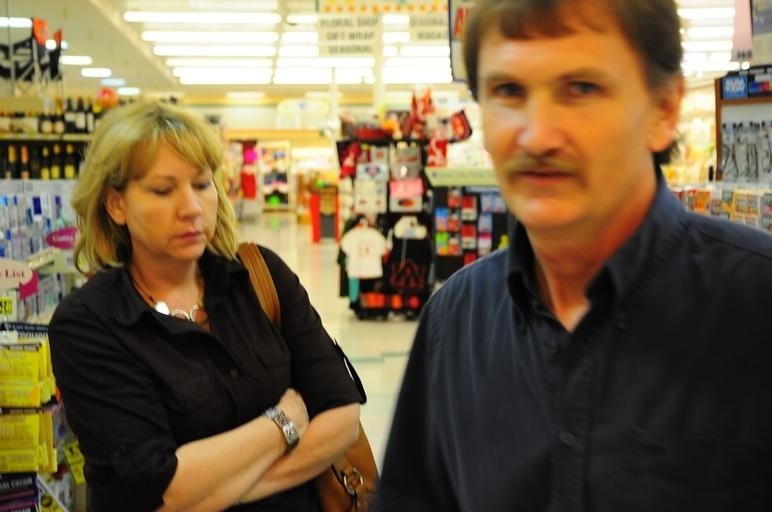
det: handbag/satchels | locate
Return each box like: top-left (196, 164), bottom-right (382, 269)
top-left (316, 421), bottom-right (378, 512)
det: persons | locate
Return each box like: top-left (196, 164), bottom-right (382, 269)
top-left (48, 99), bottom-right (366, 512)
top-left (367, 0), bottom-right (772, 512)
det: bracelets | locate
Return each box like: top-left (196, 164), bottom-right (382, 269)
top-left (232, 498), bottom-right (240, 507)
top-left (262, 407), bottom-right (299, 455)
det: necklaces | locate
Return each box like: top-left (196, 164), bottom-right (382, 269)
top-left (130, 275), bottom-right (210, 325)
